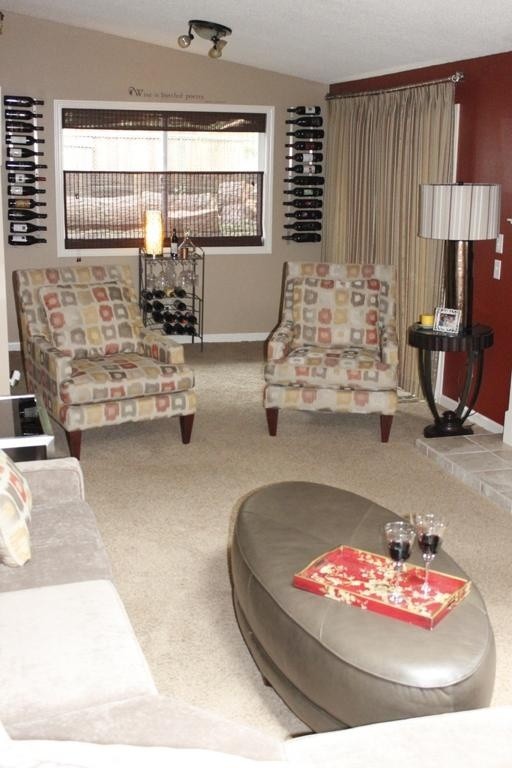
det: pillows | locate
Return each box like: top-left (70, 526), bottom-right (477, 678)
top-left (0, 461), bottom-right (33, 567)
top-left (291, 277), bottom-right (381, 352)
top-left (38, 284), bottom-right (145, 357)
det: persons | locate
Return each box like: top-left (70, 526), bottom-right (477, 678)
top-left (440, 314), bottom-right (455, 326)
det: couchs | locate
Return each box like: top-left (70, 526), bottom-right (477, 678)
top-left (0, 457), bottom-right (500, 768)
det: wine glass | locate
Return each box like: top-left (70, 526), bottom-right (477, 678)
top-left (385, 514), bottom-right (445, 597)
top-left (146, 259), bottom-right (198, 288)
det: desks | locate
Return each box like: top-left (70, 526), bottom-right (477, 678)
top-left (1, 389), bottom-right (56, 459)
top-left (405, 326), bottom-right (495, 438)
top-left (225, 480), bottom-right (496, 737)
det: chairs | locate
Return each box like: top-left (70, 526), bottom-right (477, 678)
top-left (261, 261), bottom-right (400, 443)
top-left (11, 263), bottom-right (198, 461)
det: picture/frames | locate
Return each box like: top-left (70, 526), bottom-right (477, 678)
top-left (434, 307), bottom-right (461, 333)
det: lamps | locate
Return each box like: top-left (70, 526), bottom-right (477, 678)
top-left (417, 184), bottom-right (501, 330)
top-left (143, 210), bottom-right (165, 263)
top-left (175, 19), bottom-right (234, 59)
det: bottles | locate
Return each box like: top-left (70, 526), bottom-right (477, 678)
top-left (4, 94), bottom-right (47, 245)
top-left (169, 228), bottom-right (179, 258)
top-left (281, 107), bottom-right (324, 243)
top-left (142, 289), bottom-right (197, 336)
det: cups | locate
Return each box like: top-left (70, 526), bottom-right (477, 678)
top-left (421, 314), bottom-right (433, 326)
